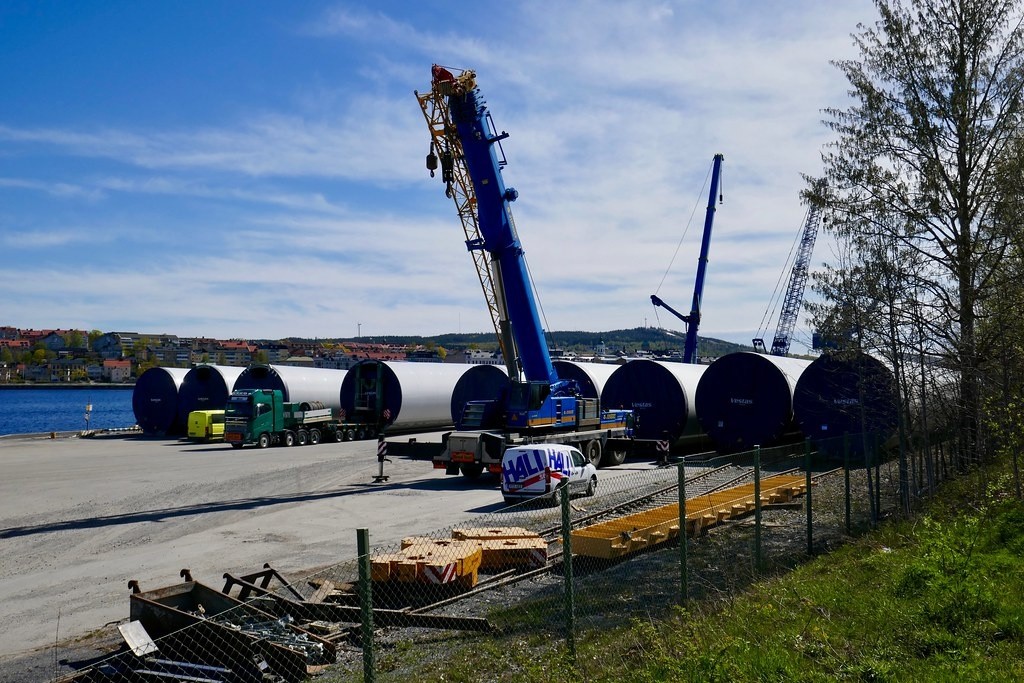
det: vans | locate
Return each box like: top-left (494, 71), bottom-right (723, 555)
top-left (188, 409), bottom-right (239, 444)
top-left (501, 444), bottom-right (598, 507)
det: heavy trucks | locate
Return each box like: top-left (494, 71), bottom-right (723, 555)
top-left (223, 389), bottom-right (378, 449)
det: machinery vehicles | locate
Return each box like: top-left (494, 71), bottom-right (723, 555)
top-left (415, 60), bottom-right (633, 436)
